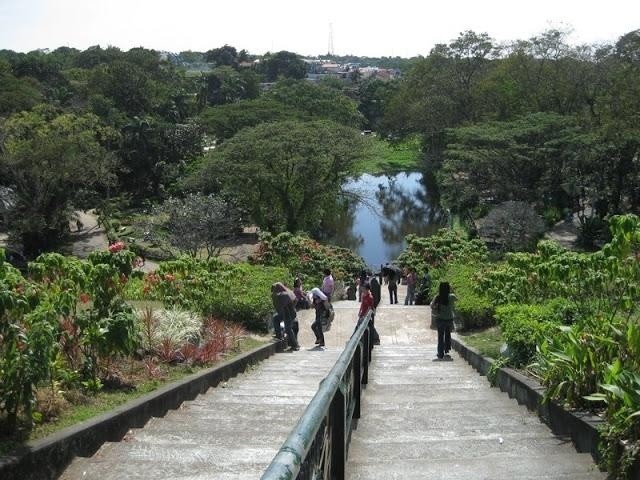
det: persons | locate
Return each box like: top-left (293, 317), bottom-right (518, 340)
top-left (270, 263), bottom-right (432, 351)
top-left (430, 281), bottom-right (458, 359)
